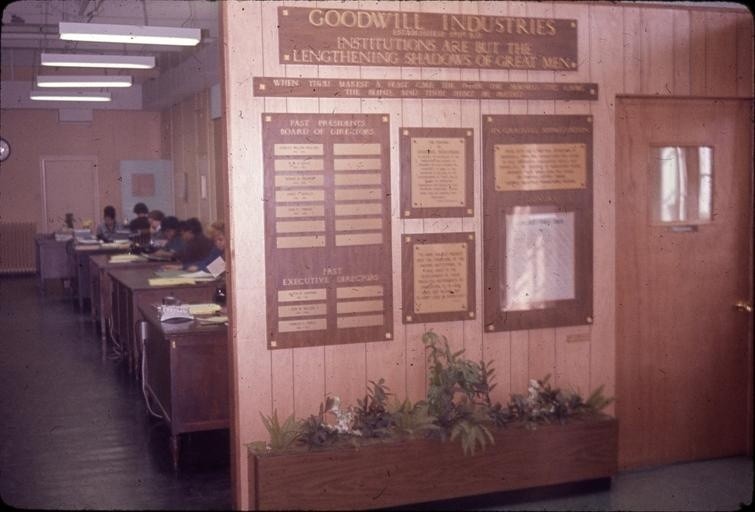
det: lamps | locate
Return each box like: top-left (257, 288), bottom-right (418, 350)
top-left (27, 2), bottom-right (202, 104)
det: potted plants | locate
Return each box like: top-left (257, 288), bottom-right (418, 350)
top-left (243, 330), bottom-right (617, 510)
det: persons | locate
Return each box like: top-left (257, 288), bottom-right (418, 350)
top-left (95, 202), bottom-right (224, 273)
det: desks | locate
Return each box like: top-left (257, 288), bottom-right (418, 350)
top-left (37, 221), bottom-right (230, 483)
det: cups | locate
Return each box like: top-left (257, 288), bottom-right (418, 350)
top-left (163, 296), bottom-right (177, 305)
